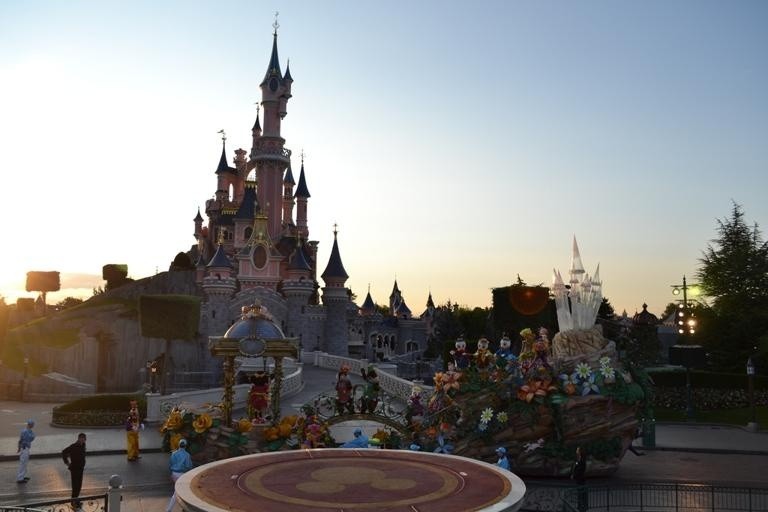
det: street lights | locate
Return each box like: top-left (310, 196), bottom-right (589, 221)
top-left (669, 273), bottom-right (700, 338)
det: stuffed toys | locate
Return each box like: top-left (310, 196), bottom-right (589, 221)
top-left (449, 336), bottom-right (471, 373)
top-left (248, 371), bottom-right (275, 424)
top-left (360, 363), bottom-right (383, 417)
top-left (335, 366), bottom-right (355, 416)
top-left (126, 399), bottom-right (145, 462)
top-left (405, 385), bottom-right (427, 419)
top-left (472, 334), bottom-right (494, 374)
top-left (495, 335), bottom-right (518, 372)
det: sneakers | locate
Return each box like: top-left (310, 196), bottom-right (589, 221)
top-left (128, 456), bottom-right (142, 462)
top-left (17, 477), bottom-right (31, 483)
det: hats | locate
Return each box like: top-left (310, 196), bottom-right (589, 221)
top-left (352, 427), bottom-right (361, 433)
top-left (495, 447), bottom-right (506, 454)
top-left (410, 444), bottom-right (420, 450)
top-left (27, 420), bottom-right (34, 424)
top-left (179, 439), bottom-right (187, 447)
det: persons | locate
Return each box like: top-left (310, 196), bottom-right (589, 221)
top-left (166, 439), bottom-right (194, 512)
top-left (490, 446), bottom-right (511, 473)
top-left (408, 431), bottom-right (422, 452)
top-left (16, 419), bottom-right (36, 483)
top-left (60, 433), bottom-right (88, 508)
top-left (569, 443), bottom-right (589, 512)
top-left (337, 428), bottom-right (368, 448)
